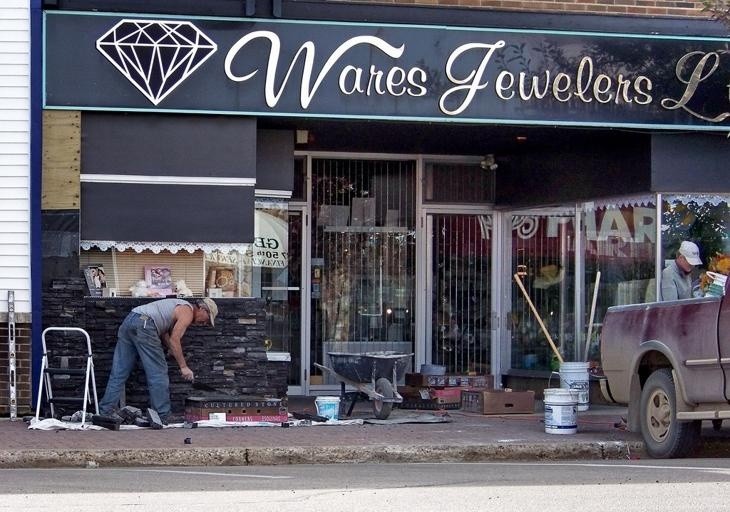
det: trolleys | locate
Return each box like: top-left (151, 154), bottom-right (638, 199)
top-left (311, 346), bottom-right (414, 419)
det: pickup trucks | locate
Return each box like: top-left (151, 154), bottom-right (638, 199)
top-left (597, 270), bottom-right (730, 459)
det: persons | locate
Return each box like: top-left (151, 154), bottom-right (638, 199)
top-left (662, 240), bottom-right (703, 303)
top-left (94, 292), bottom-right (220, 428)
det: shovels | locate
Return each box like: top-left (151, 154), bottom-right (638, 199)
top-left (178, 369), bottom-right (218, 392)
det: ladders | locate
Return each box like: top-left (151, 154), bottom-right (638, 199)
top-left (35, 327), bottom-right (99, 428)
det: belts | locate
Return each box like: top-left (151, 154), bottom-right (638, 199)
top-left (130, 311), bottom-right (154, 323)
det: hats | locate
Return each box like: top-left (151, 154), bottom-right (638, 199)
top-left (679, 240), bottom-right (703, 266)
top-left (202, 296), bottom-right (219, 327)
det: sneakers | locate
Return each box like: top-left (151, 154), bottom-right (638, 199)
top-left (110, 411), bottom-right (124, 422)
top-left (161, 414), bottom-right (184, 423)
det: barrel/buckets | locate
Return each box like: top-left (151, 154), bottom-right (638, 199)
top-left (559, 362), bottom-right (591, 411)
top-left (315, 395), bottom-right (340, 420)
top-left (543, 370), bottom-right (579, 434)
top-left (522, 354), bottom-right (537, 370)
